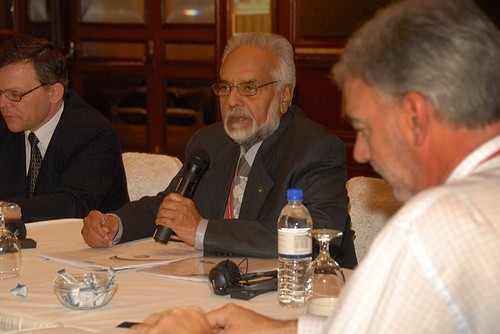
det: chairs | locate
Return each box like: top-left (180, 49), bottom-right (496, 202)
top-left (346, 176), bottom-right (404, 263)
top-left (121, 151), bottom-right (183, 202)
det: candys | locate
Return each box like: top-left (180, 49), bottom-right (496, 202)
top-left (56, 268), bottom-right (119, 308)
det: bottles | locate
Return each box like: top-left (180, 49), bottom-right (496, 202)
top-left (276, 189), bottom-right (313, 306)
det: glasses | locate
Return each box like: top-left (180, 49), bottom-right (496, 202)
top-left (0, 84), bottom-right (46, 102)
top-left (212, 81), bottom-right (278, 96)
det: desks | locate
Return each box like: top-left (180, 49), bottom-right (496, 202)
top-left (0, 217), bottom-right (355, 334)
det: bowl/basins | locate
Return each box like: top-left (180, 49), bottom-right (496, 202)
top-left (55, 272), bottom-right (118, 310)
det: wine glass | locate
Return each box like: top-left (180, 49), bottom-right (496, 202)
top-left (0, 201), bottom-right (21, 279)
top-left (306, 228), bottom-right (345, 318)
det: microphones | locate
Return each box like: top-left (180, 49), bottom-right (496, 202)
top-left (152, 146), bottom-right (210, 243)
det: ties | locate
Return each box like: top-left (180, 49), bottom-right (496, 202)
top-left (223, 155), bottom-right (252, 220)
top-left (24, 132), bottom-right (43, 199)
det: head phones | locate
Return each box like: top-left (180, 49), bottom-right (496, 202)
top-left (210, 261), bottom-right (278, 302)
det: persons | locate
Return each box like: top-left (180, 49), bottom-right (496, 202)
top-left (0, 36), bottom-right (129, 224)
top-left (129, 0), bottom-right (500, 334)
top-left (80, 32), bottom-right (349, 263)
